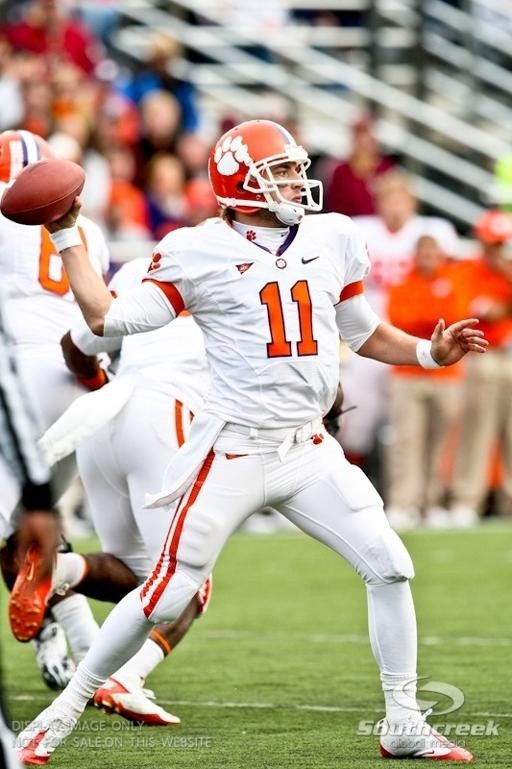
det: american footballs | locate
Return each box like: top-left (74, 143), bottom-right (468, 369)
top-left (0, 158), bottom-right (86, 226)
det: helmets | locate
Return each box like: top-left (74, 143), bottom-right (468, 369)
top-left (207, 120), bottom-right (325, 228)
top-left (0, 127), bottom-right (57, 187)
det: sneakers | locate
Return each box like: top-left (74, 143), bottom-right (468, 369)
top-left (16, 705), bottom-right (71, 765)
top-left (378, 725), bottom-right (474, 762)
top-left (91, 674), bottom-right (178, 726)
top-left (8, 541), bottom-right (74, 689)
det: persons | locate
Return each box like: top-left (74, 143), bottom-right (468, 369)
top-left (1, 0), bottom-right (512, 768)
